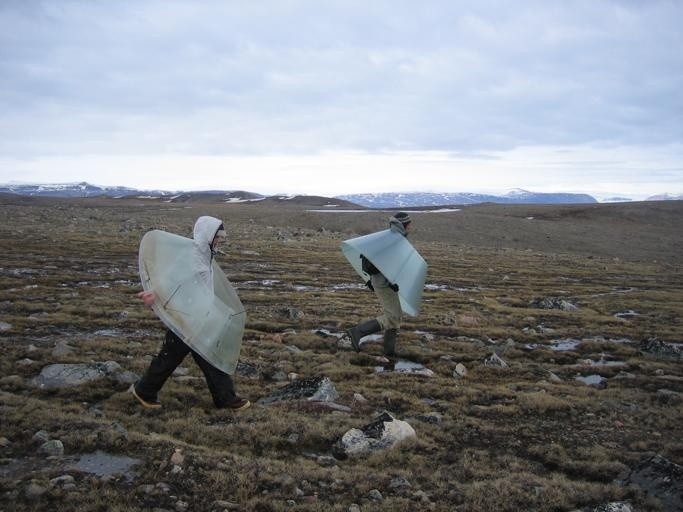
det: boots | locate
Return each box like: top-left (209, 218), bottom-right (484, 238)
top-left (383, 329), bottom-right (399, 357)
top-left (347, 320), bottom-right (381, 353)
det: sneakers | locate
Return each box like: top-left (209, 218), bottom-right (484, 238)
top-left (133, 385), bottom-right (162, 408)
top-left (219, 399), bottom-right (250, 411)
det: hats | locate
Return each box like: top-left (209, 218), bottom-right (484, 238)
top-left (392, 212), bottom-right (410, 227)
top-left (217, 225), bottom-right (227, 238)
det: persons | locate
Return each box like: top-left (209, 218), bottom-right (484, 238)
top-left (341, 211), bottom-right (429, 362)
top-left (126, 215), bottom-right (251, 412)
